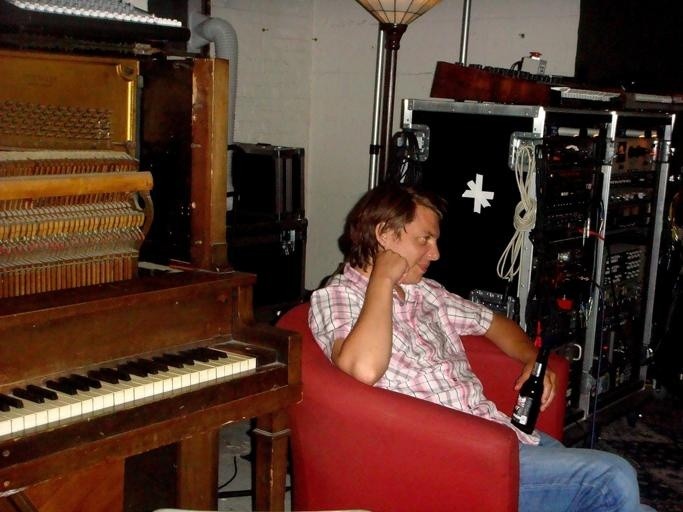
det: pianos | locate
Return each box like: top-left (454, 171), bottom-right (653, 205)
top-left (429, 60), bottom-right (626, 110)
top-left (625, 86), bottom-right (682, 114)
top-left (1, 269), bottom-right (303, 512)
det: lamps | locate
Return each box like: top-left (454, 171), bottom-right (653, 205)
top-left (350, 0), bottom-right (442, 183)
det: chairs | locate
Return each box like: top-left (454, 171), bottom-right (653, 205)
top-left (277, 301), bottom-right (569, 512)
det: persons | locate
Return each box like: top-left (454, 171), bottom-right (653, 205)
top-left (307, 182), bottom-right (661, 510)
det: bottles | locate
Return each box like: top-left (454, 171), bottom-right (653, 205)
top-left (509, 345), bottom-right (552, 435)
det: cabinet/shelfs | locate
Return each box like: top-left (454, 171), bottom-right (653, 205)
top-left (227, 140), bottom-right (309, 320)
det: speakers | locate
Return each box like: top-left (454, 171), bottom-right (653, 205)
top-left (226, 218), bottom-right (308, 324)
